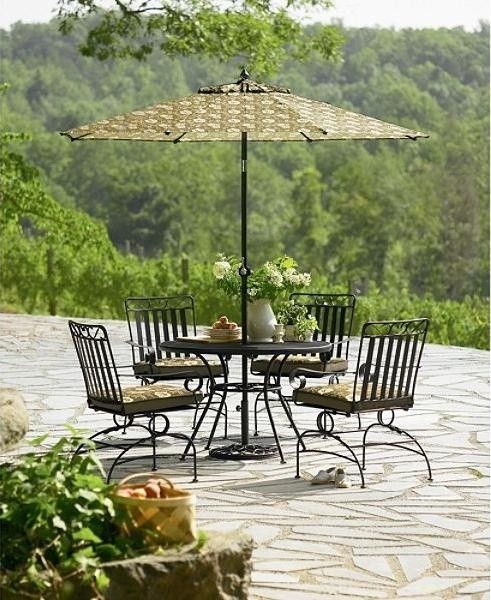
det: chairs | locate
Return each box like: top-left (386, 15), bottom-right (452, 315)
top-left (252, 292), bottom-right (357, 442)
top-left (123, 296), bottom-right (234, 439)
top-left (66, 319), bottom-right (204, 487)
top-left (292, 315), bottom-right (432, 484)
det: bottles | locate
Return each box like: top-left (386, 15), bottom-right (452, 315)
top-left (275, 324), bottom-right (286, 344)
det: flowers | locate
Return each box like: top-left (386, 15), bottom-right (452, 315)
top-left (297, 312), bottom-right (322, 334)
top-left (213, 251), bottom-right (312, 303)
top-left (277, 299), bottom-right (307, 323)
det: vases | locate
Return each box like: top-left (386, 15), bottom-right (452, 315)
top-left (303, 335), bottom-right (316, 342)
top-left (244, 298), bottom-right (280, 344)
top-left (286, 322), bottom-right (299, 341)
top-left (274, 323), bottom-right (285, 344)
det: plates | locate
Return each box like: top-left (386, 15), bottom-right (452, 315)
top-left (203, 327), bottom-right (241, 339)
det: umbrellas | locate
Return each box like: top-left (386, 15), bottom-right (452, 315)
top-left (59, 68), bottom-right (430, 445)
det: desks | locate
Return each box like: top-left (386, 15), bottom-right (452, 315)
top-left (159, 336), bottom-right (332, 464)
